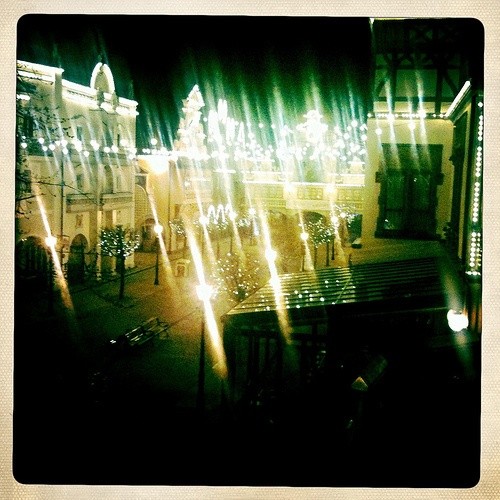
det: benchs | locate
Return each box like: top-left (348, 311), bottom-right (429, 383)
top-left (108, 318), bottom-right (171, 355)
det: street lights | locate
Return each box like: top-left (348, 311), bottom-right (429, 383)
top-left (152, 224), bottom-right (164, 285)
